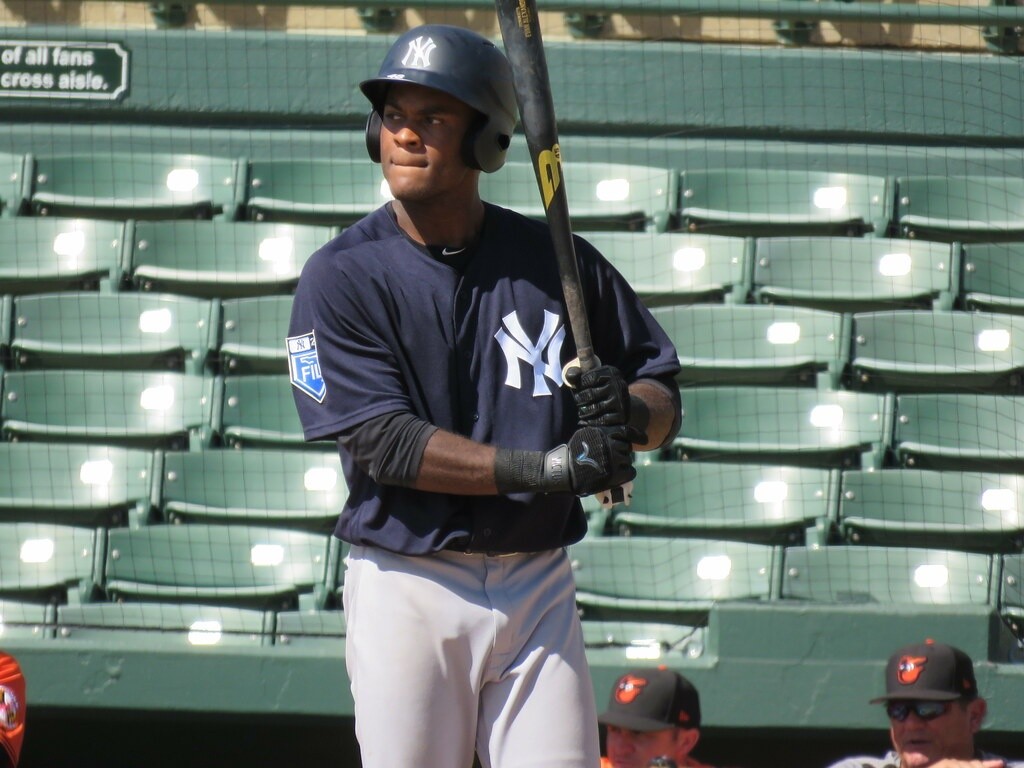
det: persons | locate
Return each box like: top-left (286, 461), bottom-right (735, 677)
top-left (828, 638), bottom-right (1024, 768)
top-left (283, 25), bottom-right (682, 768)
top-left (598, 666), bottom-right (711, 768)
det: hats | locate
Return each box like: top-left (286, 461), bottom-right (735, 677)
top-left (596, 666), bottom-right (702, 733)
top-left (868, 638), bottom-right (980, 704)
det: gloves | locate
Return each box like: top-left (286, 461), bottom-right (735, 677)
top-left (494, 426), bottom-right (649, 498)
top-left (566, 364), bottom-right (649, 438)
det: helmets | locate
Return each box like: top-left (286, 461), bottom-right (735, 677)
top-left (359, 25), bottom-right (515, 173)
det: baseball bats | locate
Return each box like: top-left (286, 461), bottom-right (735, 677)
top-left (485, 3), bottom-right (641, 508)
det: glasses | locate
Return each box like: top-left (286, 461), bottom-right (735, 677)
top-left (885, 699), bottom-right (950, 720)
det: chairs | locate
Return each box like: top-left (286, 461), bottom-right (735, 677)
top-left (0, 442), bottom-right (154, 528)
top-left (153, 449), bottom-right (349, 523)
top-left (236, 156), bottom-right (390, 225)
top-left (0, 600), bottom-right (709, 662)
top-left (2, 292), bottom-right (211, 374)
top-left (772, 545), bottom-right (1001, 608)
top-left (0, 522), bottom-right (101, 604)
top-left (477, 162), bottom-right (1024, 391)
top-left (213, 375), bottom-right (339, 449)
top-left (0, 216), bottom-right (126, 293)
top-left (886, 393), bottom-right (1024, 470)
top-left (835, 468), bottom-right (1024, 533)
top-left (121, 219), bottom-right (338, 292)
top-left (0, 366), bottom-right (212, 451)
top-left (22, 152), bottom-right (237, 222)
top-left (210, 295), bottom-right (293, 374)
top-left (564, 538), bottom-right (777, 612)
top-left (609, 462), bottom-right (836, 545)
top-left (671, 390), bottom-right (887, 468)
top-left (99, 524), bottom-right (331, 610)
